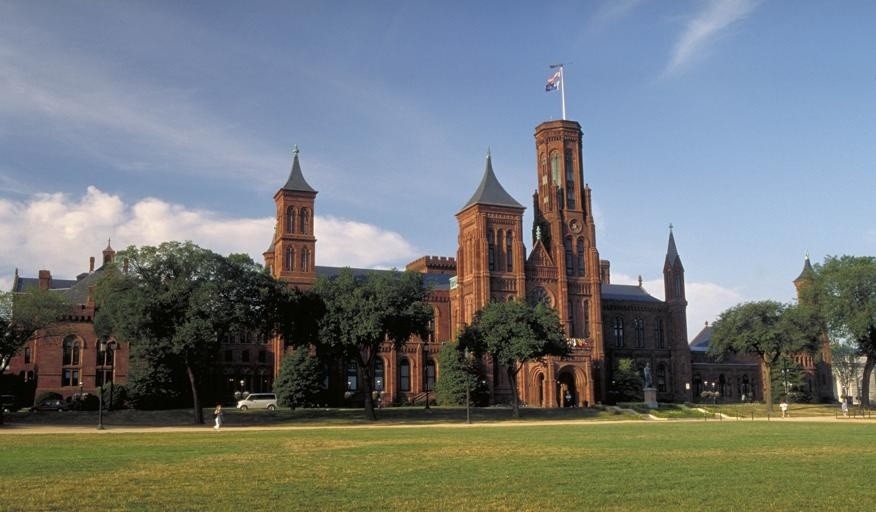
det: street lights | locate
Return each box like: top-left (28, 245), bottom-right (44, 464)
top-left (462, 345), bottom-right (472, 423)
top-left (377, 380), bottom-right (382, 408)
top-left (79, 381), bottom-right (84, 398)
top-left (840, 366), bottom-right (853, 416)
top-left (240, 379), bottom-right (245, 399)
top-left (704, 380), bottom-right (717, 407)
top-left (781, 357), bottom-right (790, 403)
top-left (96, 338), bottom-right (107, 429)
top-left (347, 381), bottom-right (352, 408)
top-left (422, 342), bottom-right (434, 409)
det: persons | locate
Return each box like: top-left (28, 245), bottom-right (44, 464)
top-left (643, 362), bottom-right (653, 388)
top-left (780, 400), bottom-right (789, 417)
top-left (213, 405), bottom-right (223, 429)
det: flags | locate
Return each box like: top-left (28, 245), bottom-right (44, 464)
top-left (544, 72), bottom-right (562, 94)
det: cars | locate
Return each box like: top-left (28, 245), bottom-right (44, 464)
top-left (29, 399), bottom-right (68, 412)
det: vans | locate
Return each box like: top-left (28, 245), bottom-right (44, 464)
top-left (1, 394), bottom-right (18, 414)
top-left (236, 392), bottom-right (277, 412)
